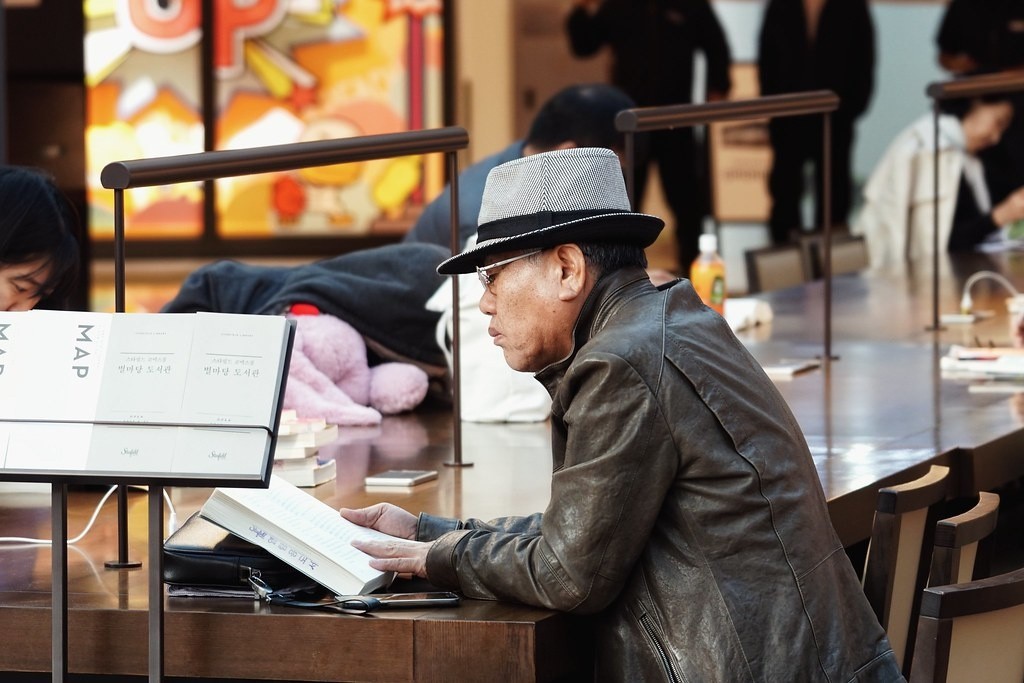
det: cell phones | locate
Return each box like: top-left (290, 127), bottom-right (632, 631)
top-left (334, 592), bottom-right (460, 611)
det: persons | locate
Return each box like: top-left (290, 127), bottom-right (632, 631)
top-left (0, 163), bottom-right (76, 319)
top-left (336, 148), bottom-right (913, 683)
top-left (405, 0), bottom-right (1024, 273)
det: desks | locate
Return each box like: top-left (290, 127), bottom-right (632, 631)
top-left (327, 231), bottom-right (1023, 551)
top-left (0, 442), bottom-right (958, 683)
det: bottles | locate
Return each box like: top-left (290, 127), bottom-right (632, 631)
top-left (691, 235), bottom-right (725, 316)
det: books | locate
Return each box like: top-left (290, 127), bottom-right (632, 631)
top-left (2, 311), bottom-right (292, 480)
top-left (197, 413), bottom-right (402, 599)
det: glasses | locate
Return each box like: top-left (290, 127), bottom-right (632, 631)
top-left (476, 247), bottom-right (554, 289)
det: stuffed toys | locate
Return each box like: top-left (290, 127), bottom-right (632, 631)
top-left (279, 311), bottom-right (431, 426)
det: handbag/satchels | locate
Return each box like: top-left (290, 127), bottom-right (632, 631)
top-left (162, 510), bottom-right (380, 615)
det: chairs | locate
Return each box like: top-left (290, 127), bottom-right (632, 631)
top-left (861, 465), bottom-right (1024, 683)
top-left (745, 226), bottom-right (869, 294)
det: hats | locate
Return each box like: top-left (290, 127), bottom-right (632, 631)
top-left (436, 147), bottom-right (664, 275)
